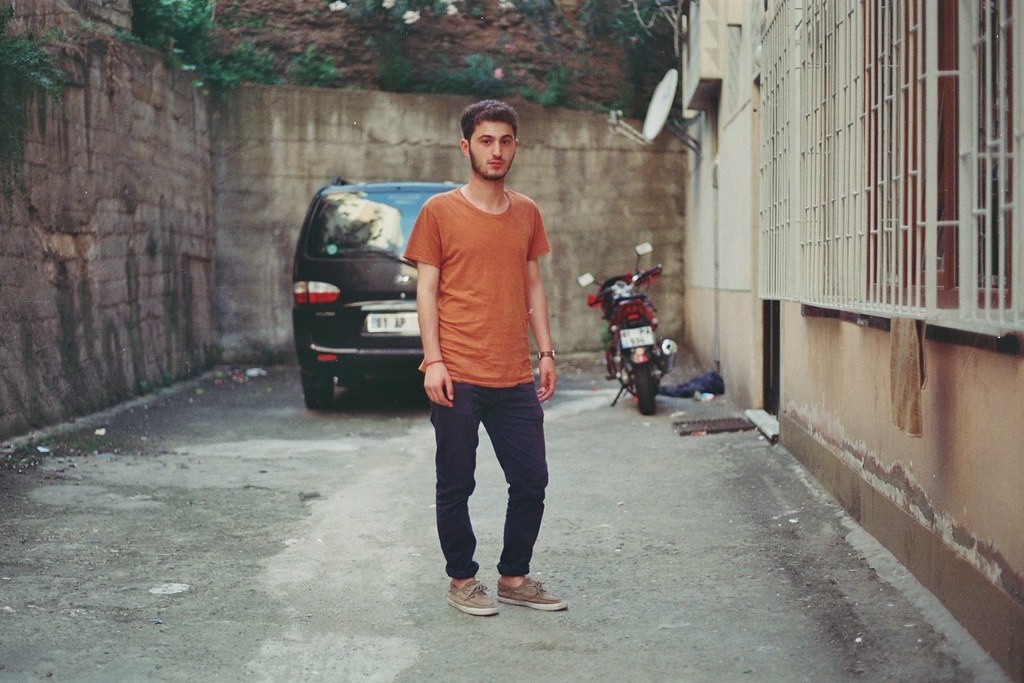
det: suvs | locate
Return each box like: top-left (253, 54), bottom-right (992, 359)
top-left (292, 177), bottom-right (468, 410)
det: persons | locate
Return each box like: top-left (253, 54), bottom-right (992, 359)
top-left (402, 98), bottom-right (569, 620)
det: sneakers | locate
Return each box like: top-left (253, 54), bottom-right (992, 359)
top-left (446, 576), bottom-right (499, 615)
top-left (497, 575), bottom-right (569, 611)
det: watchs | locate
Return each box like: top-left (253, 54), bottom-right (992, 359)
top-left (537, 349), bottom-right (555, 361)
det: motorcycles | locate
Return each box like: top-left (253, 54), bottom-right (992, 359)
top-left (577, 241), bottom-right (678, 415)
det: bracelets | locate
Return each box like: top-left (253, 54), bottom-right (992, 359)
top-left (425, 360), bottom-right (444, 366)
